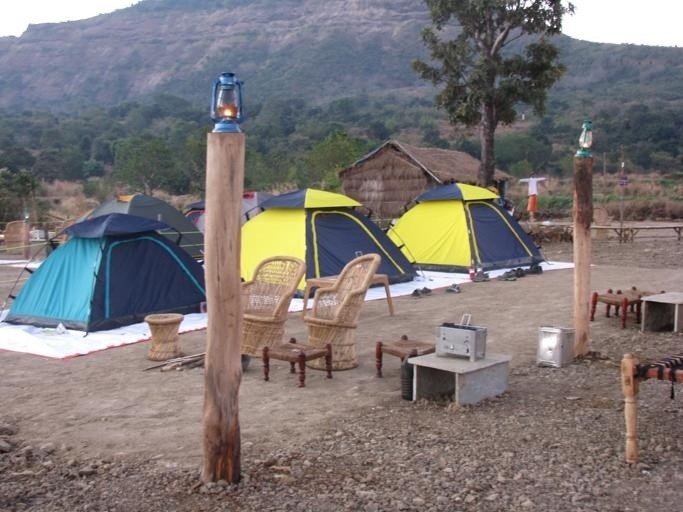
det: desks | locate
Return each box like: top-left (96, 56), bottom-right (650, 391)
top-left (301, 273), bottom-right (395, 322)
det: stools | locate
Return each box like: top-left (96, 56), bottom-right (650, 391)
top-left (143, 314), bottom-right (184, 361)
top-left (374, 335), bottom-right (436, 384)
top-left (262, 338), bottom-right (332, 388)
top-left (588, 286), bottom-right (666, 330)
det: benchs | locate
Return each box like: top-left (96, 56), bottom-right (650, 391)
top-left (527, 220), bottom-right (682, 247)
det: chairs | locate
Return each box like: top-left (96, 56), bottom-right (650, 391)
top-left (240, 252), bottom-right (382, 371)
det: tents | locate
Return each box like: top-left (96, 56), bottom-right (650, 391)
top-left (76, 189), bottom-right (205, 256)
top-left (184, 191), bottom-right (276, 234)
top-left (386, 182), bottom-right (544, 274)
top-left (239, 188), bottom-right (417, 298)
top-left (4, 212), bottom-right (205, 332)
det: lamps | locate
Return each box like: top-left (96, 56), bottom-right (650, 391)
top-left (209, 71), bottom-right (245, 133)
top-left (575, 120), bottom-right (596, 157)
